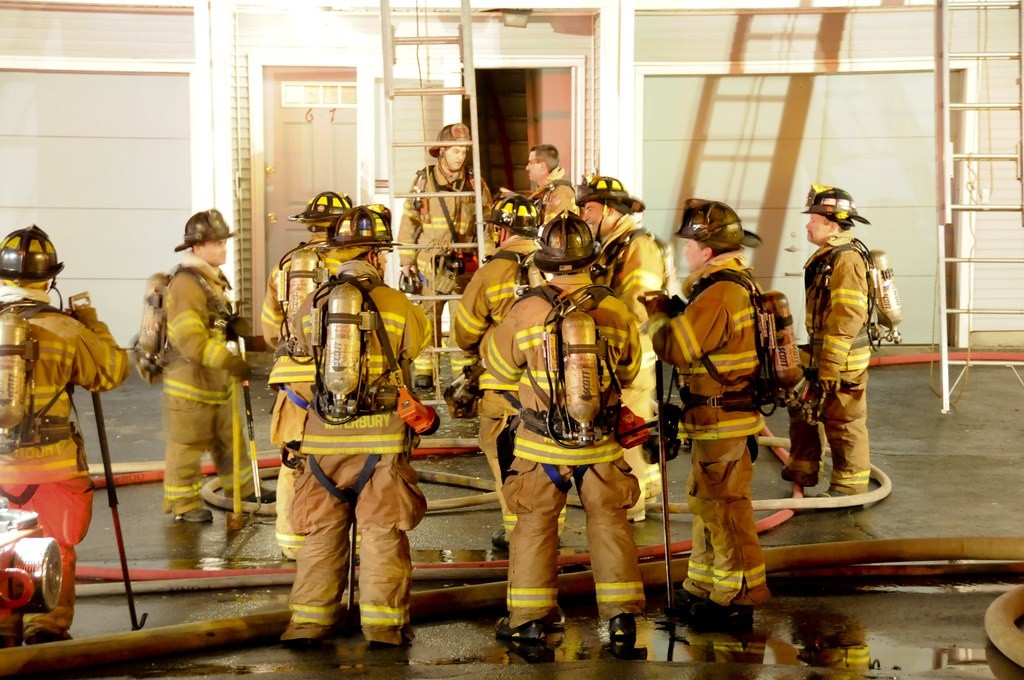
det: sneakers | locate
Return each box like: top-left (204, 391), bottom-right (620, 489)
top-left (504, 640), bottom-right (554, 664)
top-left (495, 617), bottom-right (544, 640)
top-left (609, 614), bottom-right (636, 636)
top-left (611, 637), bottom-right (635, 655)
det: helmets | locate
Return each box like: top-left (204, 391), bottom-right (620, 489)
top-left (801, 189), bottom-right (870, 226)
top-left (327, 205), bottom-right (404, 247)
top-left (575, 177), bottom-right (645, 212)
top-left (429, 124), bottom-right (471, 157)
top-left (484, 195), bottom-right (538, 240)
top-left (533, 210), bottom-right (601, 274)
top-left (288, 191), bottom-right (353, 222)
top-left (0, 225), bottom-right (64, 281)
top-left (175, 211), bottom-right (235, 251)
top-left (674, 201), bottom-right (744, 251)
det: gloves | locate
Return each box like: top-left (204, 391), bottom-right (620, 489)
top-left (818, 362), bottom-right (841, 394)
top-left (223, 354), bottom-right (252, 376)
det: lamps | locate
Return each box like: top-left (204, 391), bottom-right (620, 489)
top-left (498, 8), bottom-right (533, 28)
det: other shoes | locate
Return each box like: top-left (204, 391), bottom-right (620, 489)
top-left (371, 623), bottom-right (414, 647)
top-left (173, 508), bottom-right (213, 522)
top-left (781, 466), bottom-right (818, 487)
top-left (689, 600), bottom-right (753, 623)
top-left (816, 489), bottom-right (850, 497)
top-left (246, 487), bottom-right (277, 503)
top-left (665, 589), bottom-right (708, 610)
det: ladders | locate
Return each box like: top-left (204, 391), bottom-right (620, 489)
top-left (377, 0), bottom-right (492, 405)
top-left (929, 2), bottom-right (1024, 415)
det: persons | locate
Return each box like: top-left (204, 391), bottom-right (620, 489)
top-left (484, 208), bottom-right (642, 664)
top-left (161, 210), bottom-right (277, 522)
top-left (526, 145), bottom-right (580, 236)
top-left (398, 123), bottom-right (496, 389)
top-left (637, 197), bottom-right (772, 634)
top-left (781, 184), bottom-right (872, 498)
top-left (280, 203), bottom-right (432, 649)
top-left (454, 192), bottom-right (566, 552)
top-left (0, 223), bottom-right (129, 649)
top-left (678, 631), bottom-right (870, 669)
top-left (261, 192), bottom-right (353, 562)
top-left (574, 176), bottom-right (676, 523)
